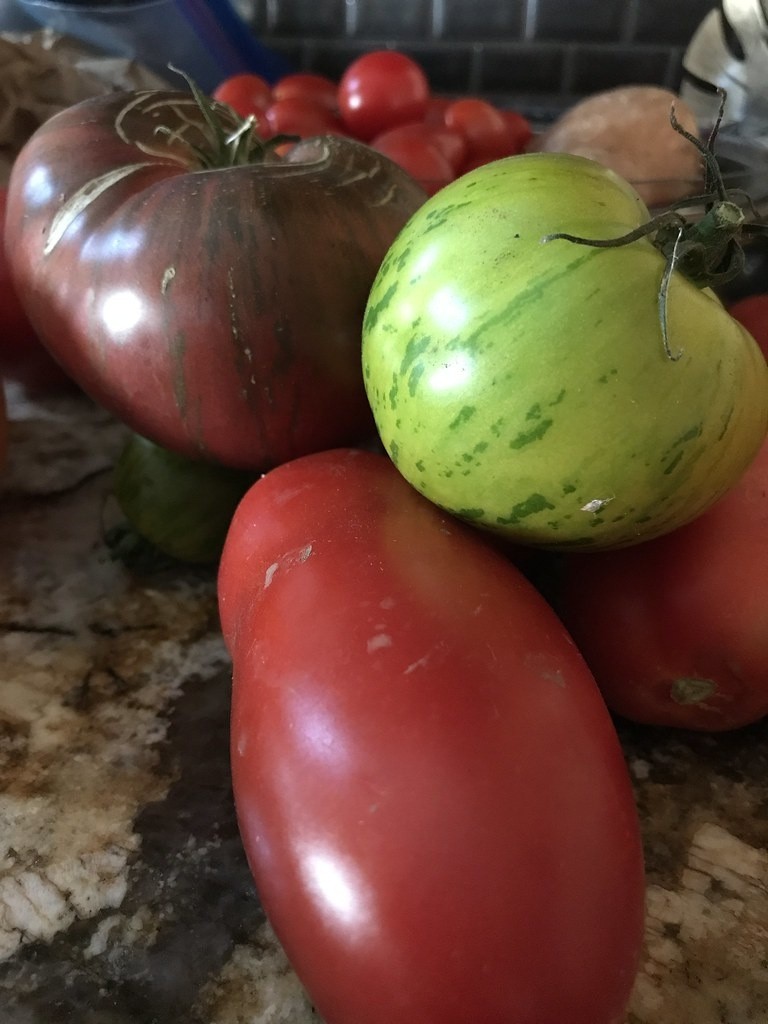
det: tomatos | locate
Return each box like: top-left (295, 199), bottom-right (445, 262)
top-left (0, 48), bottom-right (768, 1024)
